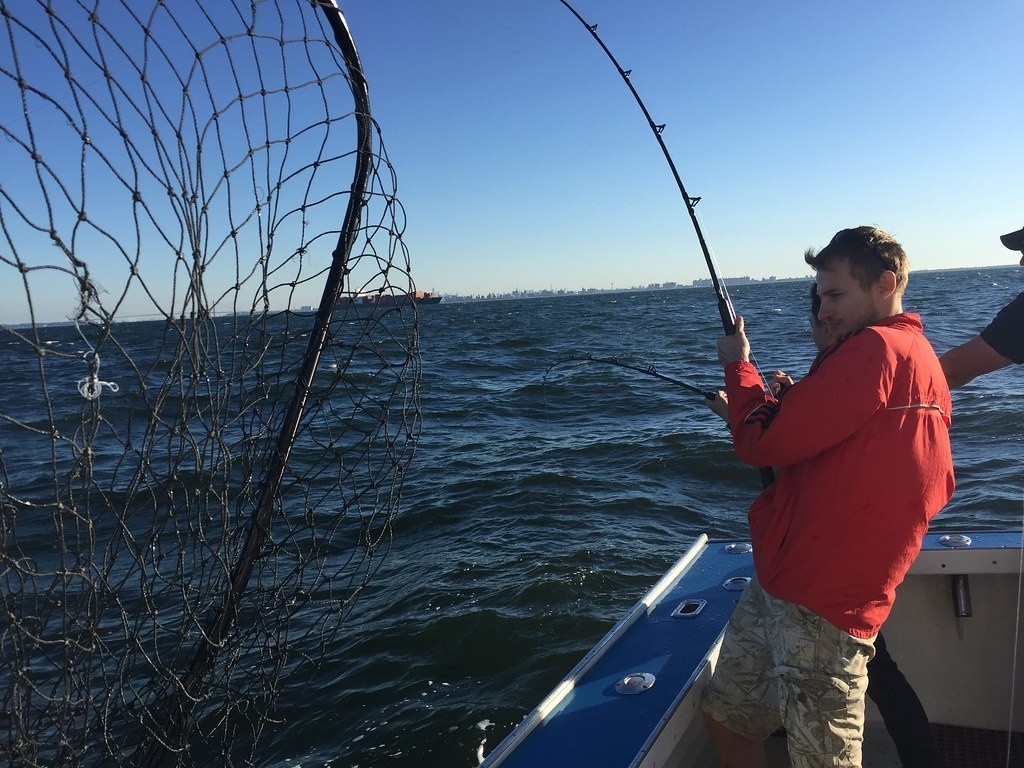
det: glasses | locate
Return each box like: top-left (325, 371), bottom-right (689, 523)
top-left (829, 230), bottom-right (893, 270)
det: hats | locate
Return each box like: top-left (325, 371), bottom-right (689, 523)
top-left (1000, 226), bottom-right (1024, 251)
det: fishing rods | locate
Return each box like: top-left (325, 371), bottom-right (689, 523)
top-left (541, 351), bottom-right (717, 401)
top-left (560, 0), bottom-right (775, 490)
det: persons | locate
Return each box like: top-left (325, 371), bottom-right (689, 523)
top-left (698, 224), bottom-right (955, 768)
top-left (938, 225), bottom-right (1023, 389)
top-left (704, 282), bottom-right (941, 768)
